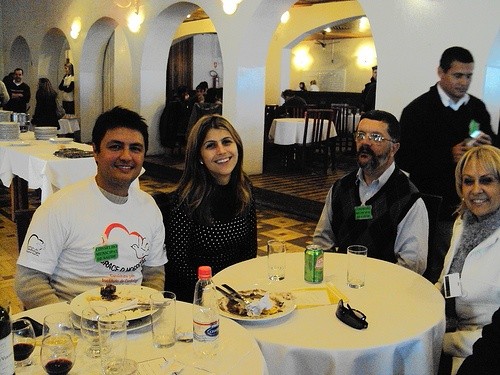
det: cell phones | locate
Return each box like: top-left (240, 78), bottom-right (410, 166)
top-left (12, 316), bottom-right (49, 337)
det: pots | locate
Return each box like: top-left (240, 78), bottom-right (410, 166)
top-left (12, 112), bottom-right (31, 132)
top-left (0, 110), bottom-right (12, 121)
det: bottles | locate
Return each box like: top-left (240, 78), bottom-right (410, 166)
top-left (192, 265), bottom-right (221, 358)
top-left (0, 306), bottom-right (15, 375)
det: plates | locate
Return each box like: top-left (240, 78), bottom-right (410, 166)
top-left (0, 121), bottom-right (21, 141)
top-left (62, 117), bottom-right (77, 119)
top-left (34, 126), bottom-right (75, 144)
top-left (69, 284), bottom-right (165, 332)
top-left (218, 285), bottom-right (297, 322)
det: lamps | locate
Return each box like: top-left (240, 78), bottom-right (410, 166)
top-left (126, 0), bottom-right (144, 33)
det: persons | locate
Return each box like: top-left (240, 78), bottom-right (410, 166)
top-left (159, 81), bottom-right (223, 114)
top-left (0, 68), bottom-right (31, 113)
top-left (162, 115), bottom-right (257, 303)
top-left (398, 46), bottom-right (491, 219)
top-left (360, 66), bottom-right (378, 109)
top-left (14, 105), bottom-right (168, 309)
top-left (308, 80), bottom-right (319, 91)
top-left (297, 82), bottom-right (307, 91)
top-left (435, 145), bottom-right (500, 375)
top-left (456, 306), bottom-right (500, 375)
top-left (310, 110), bottom-right (428, 274)
top-left (268, 89), bottom-right (308, 119)
top-left (57, 63), bottom-right (74, 115)
top-left (29, 78), bottom-right (60, 131)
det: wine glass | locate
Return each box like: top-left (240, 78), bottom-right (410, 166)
top-left (81, 305), bottom-right (111, 357)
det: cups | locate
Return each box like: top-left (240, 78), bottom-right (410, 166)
top-left (98, 312), bottom-right (138, 375)
top-left (347, 245), bottom-right (368, 288)
top-left (267, 239), bottom-right (286, 281)
top-left (39, 311), bottom-right (79, 375)
top-left (149, 291), bottom-right (177, 349)
top-left (11, 319), bottom-right (37, 361)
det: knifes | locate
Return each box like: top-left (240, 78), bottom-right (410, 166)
top-left (216, 286), bottom-right (245, 305)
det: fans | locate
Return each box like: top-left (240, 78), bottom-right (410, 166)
top-left (314, 31), bottom-right (340, 49)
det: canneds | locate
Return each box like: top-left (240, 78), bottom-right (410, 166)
top-left (304, 244), bottom-right (324, 284)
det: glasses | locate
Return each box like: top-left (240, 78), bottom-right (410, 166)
top-left (335, 298), bottom-right (370, 329)
top-left (352, 130), bottom-right (395, 146)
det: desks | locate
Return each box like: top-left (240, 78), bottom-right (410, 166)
top-left (335, 111), bottom-right (361, 133)
top-left (203, 252), bottom-right (446, 375)
top-left (0, 118), bottom-right (146, 222)
top-left (10, 298), bottom-right (268, 375)
top-left (268, 118), bottom-right (337, 174)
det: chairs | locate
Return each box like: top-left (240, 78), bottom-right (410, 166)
top-left (295, 109), bottom-right (336, 174)
top-left (331, 104), bottom-right (358, 151)
